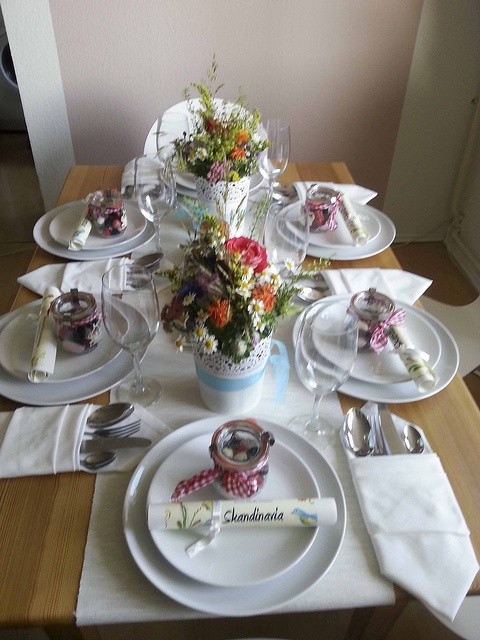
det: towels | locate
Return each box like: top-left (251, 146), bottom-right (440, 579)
top-left (287, 268), bottom-right (433, 308)
top-left (121, 157), bottom-right (165, 197)
top-left (17, 257), bottom-right (152, 297)
top-left (0, 403), bottom-right (172, 479)
top-left (340, 400), bottom-right (479, 623)
top-left (284, 181), bottom-right (378, 206)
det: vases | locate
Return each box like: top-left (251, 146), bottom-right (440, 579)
top-left (194, 177), bottom-right (250, 239)
top-left (189, 328), bottom-right (272, 414)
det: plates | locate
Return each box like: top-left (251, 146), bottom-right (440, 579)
top-left (146, 431), bottom-right (321, 588)
top-left (32, 200), bottom-right (157, 261)
top-left (0, 297), bottom-right (151, 407)
top-left (173, 172), bottom-right (196, 191)
top-left (286, 203), bottom-right (381, 249)
top-left (120, 412), bottom-right (348, 619)
top-left (310, 296), bottom-right (441, 385)
top-left (276, 199), bottom-right (397, 261)
top-left (176, 174), bottom-right (265, 199)
top-left (48, 203), bottom-right (148, 251)
top-left (0, 303), bottom-right (130, 384)
top-left (293, 294), bottom-right (460, 404)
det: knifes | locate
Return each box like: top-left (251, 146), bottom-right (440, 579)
top-left (294, 279), bottom-right (328, 290)
top-left (80, 437), bottom-right (152, 453)
top-left (378, 403), bottom-right (403, 455)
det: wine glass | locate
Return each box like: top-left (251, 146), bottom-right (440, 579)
top-left (248, 119), bottom-right (292, 205)
top-left (133, 154), bottom-right (179, 273)
top-left (263, 200), bottom-right (309, 314)
top-left (101, 264), bottom-right (163, 406)
top-left (285, 301), bottom-right (360, 453)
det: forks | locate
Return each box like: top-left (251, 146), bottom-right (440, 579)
top-left (84, 418), bottom-right (141, 439)
top-left (366, 415), bottom-right (386, 456)
top-left (295, 287), bottom-right (327, 303)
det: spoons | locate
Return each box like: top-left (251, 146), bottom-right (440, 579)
top-left (343, 406), bottom-right (375, 456)
top-left (86, 402), bottom-right (134, 428)
top-left (80, 451), bottom-right (117, 470)
top-left (136, 253), bottom-right (164, 269)
top-left (403, 425), bottom-right (424, 453)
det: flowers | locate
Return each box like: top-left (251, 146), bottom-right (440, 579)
top-left (161, 162), bottom-right (336, 364)
top-left (168, 53), bottom-right (271, 184)
